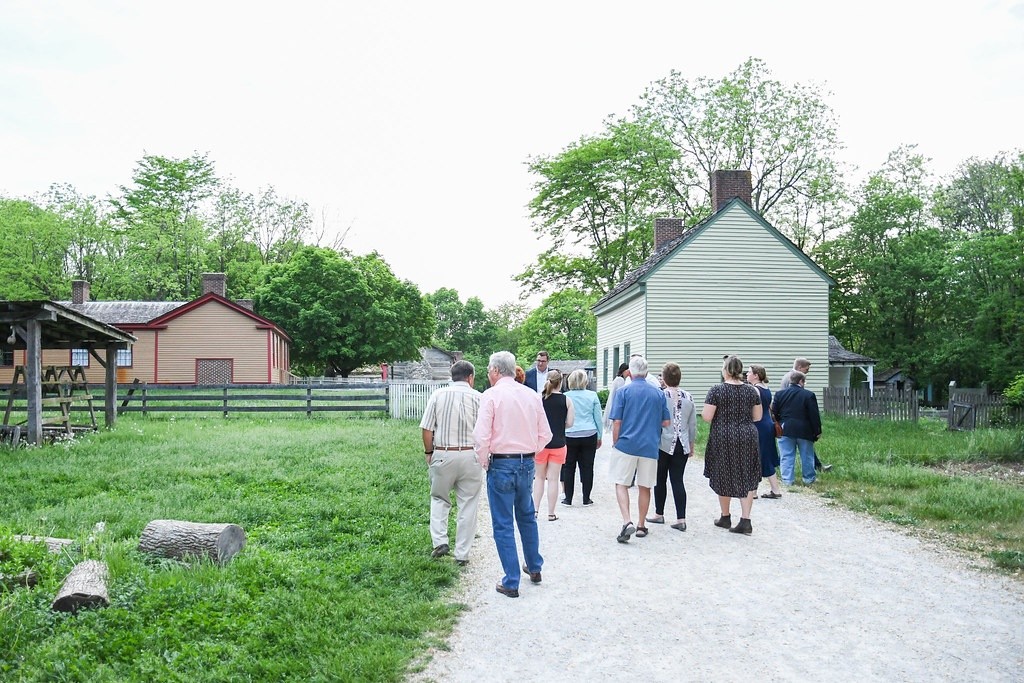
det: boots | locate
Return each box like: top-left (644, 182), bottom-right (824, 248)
top-left (730, 518), bottom-right (753, 533)
top-left (714, 513), bottom-right (732, 529)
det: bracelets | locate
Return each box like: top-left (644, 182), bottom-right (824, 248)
top-left (425, 451), bottom-right (433, 454)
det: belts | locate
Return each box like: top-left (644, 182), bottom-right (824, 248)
top-left (490, 453), bottom-right (535, 459)
top-left (436, 447), bottom-right (473, 450)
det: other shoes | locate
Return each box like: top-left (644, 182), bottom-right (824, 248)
top-left (671, 522), bottom-right (686, 531)
top-left (819, 465), bottom-right (832, 472)
top-left (646, 516), bottom-right (664, 523)
top-left (561, 499), bottom-right (571, 505)
top-left (455, 560), bottom-right (469, 565)
top-left (583, 499), bottom-right (594, 506)
top-left (560, 493), bottom-right (567, 502)
top-left (431, 544), bottom-right (449, 557)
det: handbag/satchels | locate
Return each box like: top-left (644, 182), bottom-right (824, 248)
top-left (772, 422), bottom-right (784, 437)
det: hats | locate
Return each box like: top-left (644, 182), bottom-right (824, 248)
top-left (618, 363), bottom-right (629, 376)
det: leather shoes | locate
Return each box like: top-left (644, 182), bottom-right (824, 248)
top-left (495, 581), bottom-right (519, 597)
top-left (523, 562), bottom-right (541, 582)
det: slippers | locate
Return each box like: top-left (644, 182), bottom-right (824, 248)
top-left (761, 491), bottom-right (782, 498)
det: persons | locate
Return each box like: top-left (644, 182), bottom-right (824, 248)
top-left (608, 356), bottom-right (670, 540)
top-left (780, 358), bottom-right (832, 473)
top-left (563, 370), bottom-right (603, 507)
top-left (532, 369), bottom-right (574, 520)
top-left (514, 366), bottom-right (526, 383)
top-left (420, 360), bottom-right (483, 565)
top-left (744, 365), bottom-right (782, 499)
top-left (603, 354), bottom-right (670, 488)
top-left (771, 372), bottom-right (822, 487)
top-left (647, 362), bottom-right (696, 531)
top-left (701, 355), bottom-right (763, 533)
top-left (472, 351), bottom-right (553, 598)
top-left (525, 351), bottom-right (552, 395)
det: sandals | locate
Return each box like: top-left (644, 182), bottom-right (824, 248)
top-left (617, 522), bottom-right (635, 542)
top-left (548, 515), bottom-right (559, 521)
top-left (636, 526), bottom-right (649, 537)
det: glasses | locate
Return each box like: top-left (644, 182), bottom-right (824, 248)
top-left (537, 359), bottom-right (546, 363)
top-left (723, 355), bottom-right (728, 361)
top-left (549, 369), bottom-right (561, 384)
